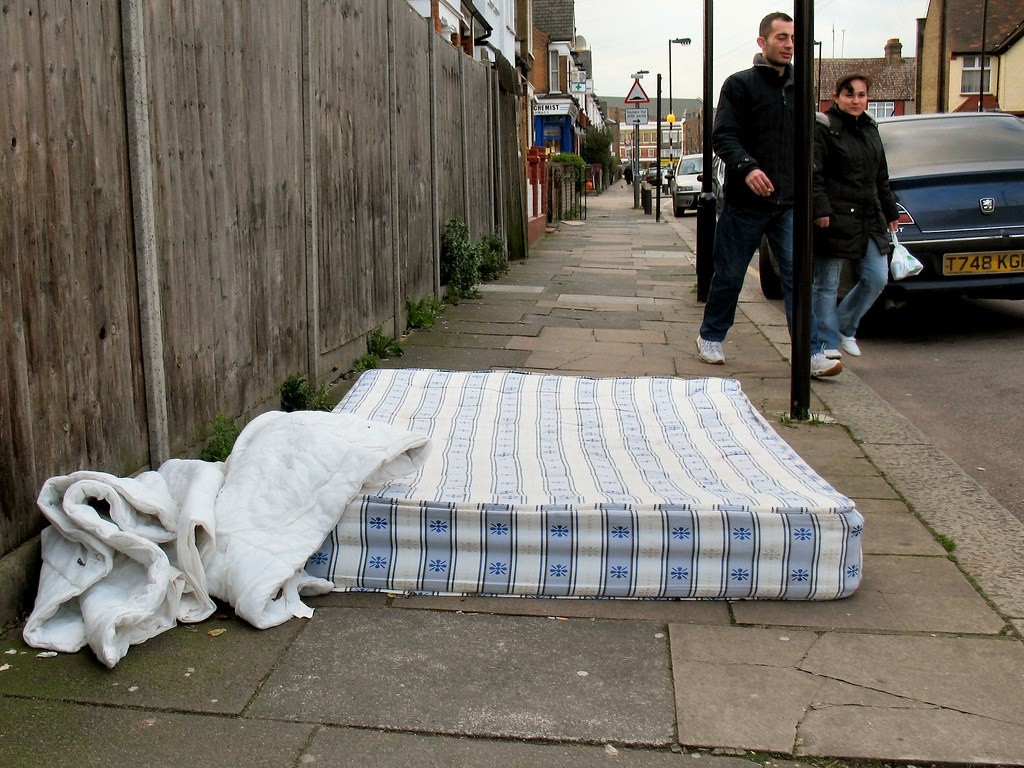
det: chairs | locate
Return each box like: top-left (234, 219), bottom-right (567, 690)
top-left (683, 161), bottom-right (698, 173)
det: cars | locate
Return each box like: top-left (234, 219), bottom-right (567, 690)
top-left (867, 111), bottom-right (1024, 314)
top-left (665, 154), bottom-right (703, 217)
top-left (639, 166), bottom-right (676, 186)
top-left (696, 153), bottom-right (725, 219)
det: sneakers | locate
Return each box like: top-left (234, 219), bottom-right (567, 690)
top-left (823, 349), bottom-right (841, 358)
top-left (694, 334), bottom-right (725, 364)
top-left (808, 343), bottom-right (842, 377)
top-left (839, 331), bottom-right (861, 356)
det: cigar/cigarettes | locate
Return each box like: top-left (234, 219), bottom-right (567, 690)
top-left (767, 188), bottom-right (771, 192)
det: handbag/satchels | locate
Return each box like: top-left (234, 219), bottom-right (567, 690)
top-left (891, 230), bottom-right (922, 281)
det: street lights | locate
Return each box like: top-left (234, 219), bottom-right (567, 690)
top-left (669, 38), bottom-right (692, 193)
top-left (813, 41), bottom-right (822, 111)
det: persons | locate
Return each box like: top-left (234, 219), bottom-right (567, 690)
top-left (623, 165), bottom-right (633, 186)
top-left (814, 72), bottom-right (900, 359)
top-left (696, 11), bottom-right (843, 377)
